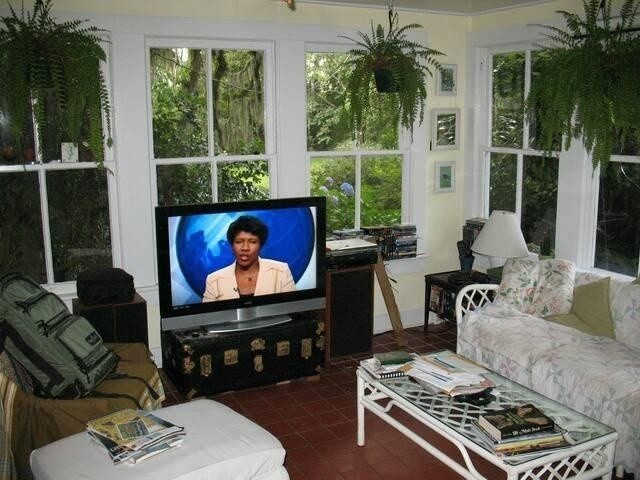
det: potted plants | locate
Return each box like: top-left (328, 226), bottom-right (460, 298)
top-left (336, 1), bottom-right (452, 146)
top-left (0, 0), bottom-right (115, 179)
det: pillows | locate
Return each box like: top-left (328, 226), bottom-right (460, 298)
top-left (530, 257), bottom-right (575, 317)
top-left (491, 251), bottom-right (540, 315)
top-left (539, 275), bottom-right (618, 342)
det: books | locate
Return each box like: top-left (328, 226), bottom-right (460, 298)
top-left (361, 346), bottom-right (568, 455)
top-left (457, 217), bottom-right (488, 256)
top-left (325, 222), bottom-right (419, 260)
top-left (84, 406), bottom-right (187, 468)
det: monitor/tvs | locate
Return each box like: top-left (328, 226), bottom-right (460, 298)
top-left (155, 196), bottom-right (326, 334)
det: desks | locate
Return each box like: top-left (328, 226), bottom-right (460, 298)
top-left (422, 268), bottom-right (488, 338)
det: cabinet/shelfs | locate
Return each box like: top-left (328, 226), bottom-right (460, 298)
top-left (159, 312), bottom-right (327, 400)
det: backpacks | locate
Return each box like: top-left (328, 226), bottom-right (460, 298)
top-left (0, 270), bottom-right (123, 403)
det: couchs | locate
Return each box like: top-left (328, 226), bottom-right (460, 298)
top-left (454, 267), bottom-right (640, 479)
top-left (0, 340), bottom-right (167, 480)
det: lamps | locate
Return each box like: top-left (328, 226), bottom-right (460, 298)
top-left (469, 208), bottom-right (530, 269)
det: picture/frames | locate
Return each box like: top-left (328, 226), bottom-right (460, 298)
top-left (432, 159), bottom-right (456, 195)
top-left (433, 62), bottom-right (459, 97)
top-left (430, 107), bottom-right (461, 152)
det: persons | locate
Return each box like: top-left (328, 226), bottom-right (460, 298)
top-left (199, 214), bottom-right (299, 307)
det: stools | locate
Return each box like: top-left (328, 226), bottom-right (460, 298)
top-left (28, 398), bottom-right (293, 480)
